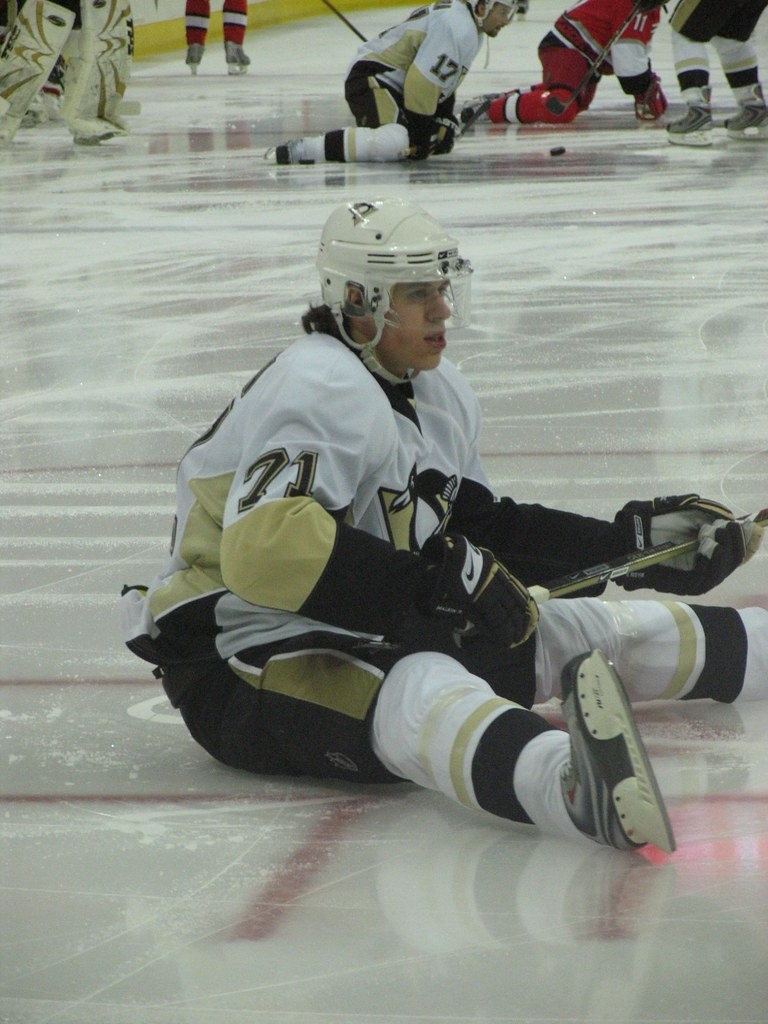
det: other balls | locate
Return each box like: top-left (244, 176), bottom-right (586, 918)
top-left (550, 146), bottom-right (565, 156)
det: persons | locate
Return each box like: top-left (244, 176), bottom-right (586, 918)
top-left (667, 0), bottom-right (768, 146)
top-left (123, 197), bottom-right (768, 851)
top-left (0, 0), bottom-right (134, 146)
top-left (265, 0), bottom-right (529, 164)
top-left (185, 0), bottom-right (251, 74)
top-left (462, 0), bottom-right (667, 126)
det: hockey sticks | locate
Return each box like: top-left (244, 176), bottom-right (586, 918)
top-left (323, 2), bottom-right (493, 140)
top-left (527, 506), bottom-right (768, 607)
top-left (543, 0), bottom-right (655, 115)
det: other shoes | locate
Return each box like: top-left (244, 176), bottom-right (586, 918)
top-left (0, 113), bottom-right (20, 148)
top-left (67, 116), bottom-right (127, 146)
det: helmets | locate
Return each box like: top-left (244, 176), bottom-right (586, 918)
top-left (317, 195), bottom-right (472, 331)
top-left (467, 0), bottom-right (529, 26)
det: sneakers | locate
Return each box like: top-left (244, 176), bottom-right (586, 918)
top-left (559, 650), bottom-right (677, 854)
top-left (225, 40), bottom-right (251, 74)
top-left (667, 86), bottom-right (713, 146)
top-left (723, 84), bottom-right (768, 140)
top-left (186, 43), bottom-right (204, 74)
top-left (461, 93), bottom-right (499, 123)
top-left (264, 138), bottom-right (315, 164)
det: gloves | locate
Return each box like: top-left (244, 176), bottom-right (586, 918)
top-left (415, 533), bottom-right (539, 676)
top-left (411, 123), bottom-right (440, 160)
top-left (433, 114), bottom-right (459, 155)
top-left (635, 73), bottom-right (668, 120)
top-left (611, 494), bottom-right (764, 596)
